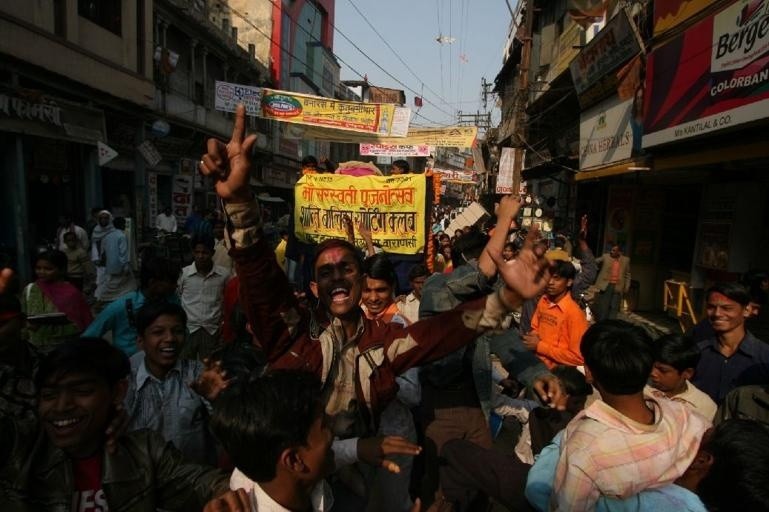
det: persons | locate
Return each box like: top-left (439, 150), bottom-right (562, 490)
top-left (198, 100), bottom-right (556, 512)
top-left (0, 154), bottom-right (769, 512)
